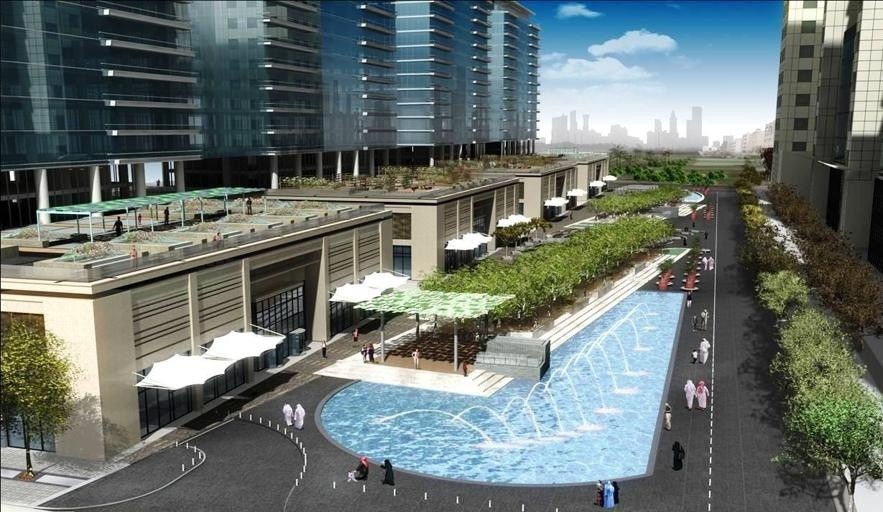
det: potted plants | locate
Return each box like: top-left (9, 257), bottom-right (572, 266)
top-left (657, 257), bottom-right (696, 292)
top-left (689, 203), bottom-right (713, 221)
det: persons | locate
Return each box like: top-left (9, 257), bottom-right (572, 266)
top-left (379, 460), bottom-right (394, 486)
top-left (569, 211), bottom-right (573, 220)
top-left (293, 403), bottom-right (305, 429)
top-left (595, 231), bottom-right (714, 508)
top-left (112, 178), bottom-right (252, 242)
top-left (321, 327), bottom-right (480, 377)
top-left (354, 457), bottom-right (368, 480)
top-left (282, 400), bottom-right (292, 425)
